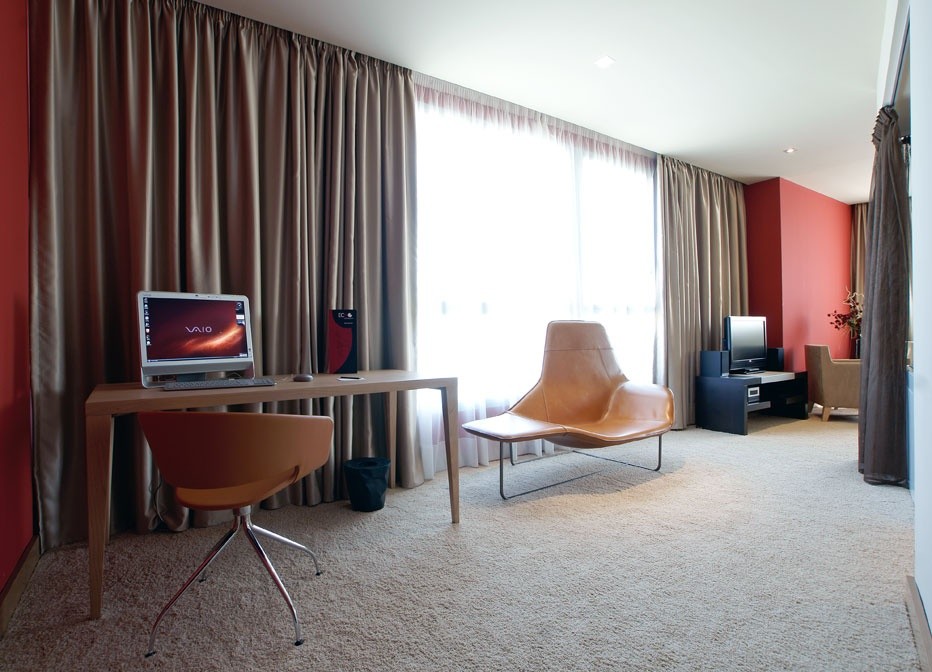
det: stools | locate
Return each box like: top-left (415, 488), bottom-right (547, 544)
top-left (134, 410), bottom-right (336, 656)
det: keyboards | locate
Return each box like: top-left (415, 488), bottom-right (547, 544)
top-left (164, 378), bottom-right (277, 390)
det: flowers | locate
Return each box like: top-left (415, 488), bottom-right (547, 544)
top-left (828, 288), bottom-right (864, 343)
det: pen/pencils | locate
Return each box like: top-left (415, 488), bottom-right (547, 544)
top-left (341, 376), bottom-right (360, 379)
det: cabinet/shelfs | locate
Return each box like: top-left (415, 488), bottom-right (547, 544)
top-left (694, 371), bottom-right (808, 435)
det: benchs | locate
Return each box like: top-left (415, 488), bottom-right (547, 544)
top-left (461, 319), bottom-right (674, 500)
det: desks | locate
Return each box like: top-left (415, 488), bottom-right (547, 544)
top-left (86, 370), bottom-right (460, 617)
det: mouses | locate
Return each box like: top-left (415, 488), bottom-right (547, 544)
top-left (294, 374), bottom-right (314, 382)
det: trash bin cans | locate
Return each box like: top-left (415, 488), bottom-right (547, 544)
top-left (344, 457), bottom-right (391, 512)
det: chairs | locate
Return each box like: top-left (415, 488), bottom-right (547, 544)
top-left (804, 344), bottom-right (861, 420)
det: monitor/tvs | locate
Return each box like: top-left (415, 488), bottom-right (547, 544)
top-left (138, 291), bottom-right (255, 388)
top-left (723, 316), bottom-right (767, 373)
